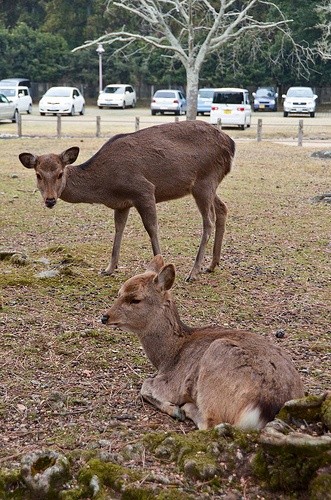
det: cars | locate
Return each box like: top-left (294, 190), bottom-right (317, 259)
top-left (1, 86), bottom-right (33, 114)
top-left (0, 78), bottom-right (33, 98)
top-left (97, 84), bottom-right (137, 109)
top-left (252, 89), bottom-right (278, 112)
top-left (196, 87), bottom-right (252, 130)
top-left (0, 91), bottom-right (20, 123)
top-left (151, 89), bottom-right (187, 116)
top-left (281, 86), bottom-right (318, 117)
top-left (39, 86), bottom-right (85, 116)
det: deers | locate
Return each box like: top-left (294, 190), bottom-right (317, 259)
top-left (101, 254), bottom-right (304, 432)
top-left (19, 120), bottom-right (236, 282)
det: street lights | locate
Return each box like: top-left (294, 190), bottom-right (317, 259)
top-left (96, 44), bottom-right (105, 94)
